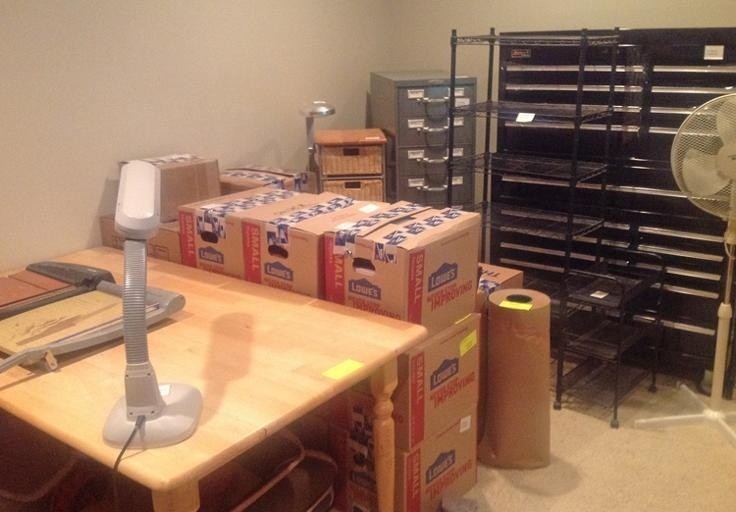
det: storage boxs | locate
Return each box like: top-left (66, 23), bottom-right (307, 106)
top-left (100, 69), bottom-right (523, 512)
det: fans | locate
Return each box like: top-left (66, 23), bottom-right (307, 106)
top-left (632, 93), bottom-right (736, 445)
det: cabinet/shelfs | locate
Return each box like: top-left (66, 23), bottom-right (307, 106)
top-left (446, 24), bottom-right (659, 354)
top-left (553, 248), bottom-right (665, 429)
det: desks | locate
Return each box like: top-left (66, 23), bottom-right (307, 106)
top-left (1, 244), bottom-right (426, 510)
